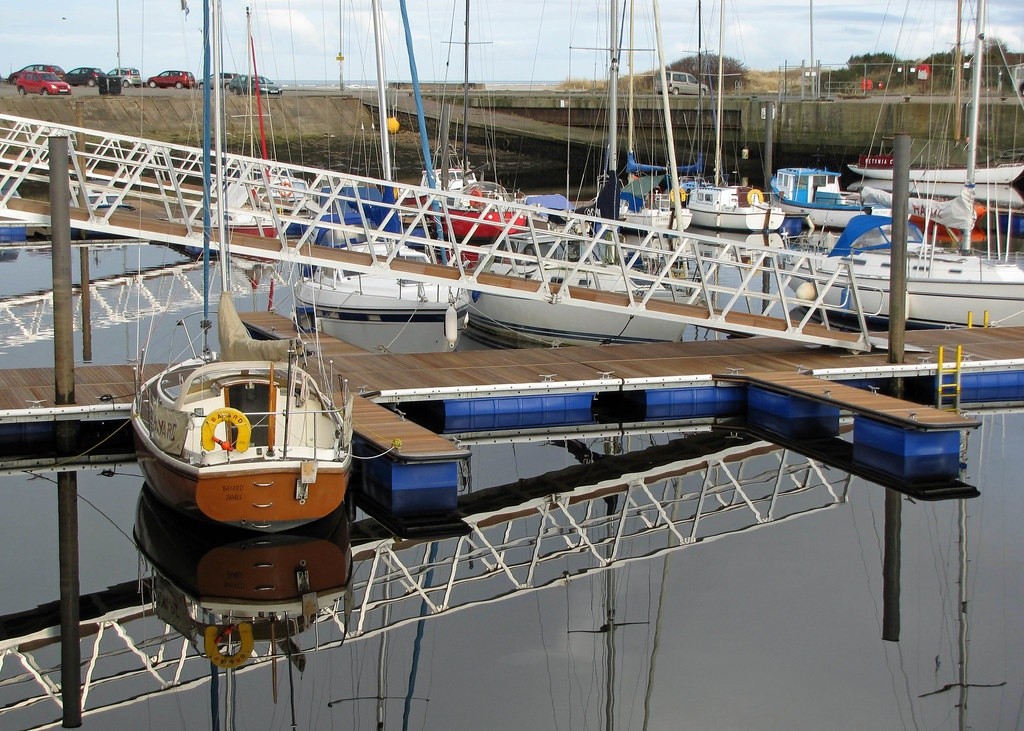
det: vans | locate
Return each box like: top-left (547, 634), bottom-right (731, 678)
top-left (229, 74), bottom-right (283, 95)
top-left (653, 70), bottom-right (708, 95)
top-left (197, 72), bottom-right (240, 90)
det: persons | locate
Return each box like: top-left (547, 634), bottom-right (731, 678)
top-left (596, 169), bottom-right (621, 265)
top-left (913, 59), bottom-right (930, 95)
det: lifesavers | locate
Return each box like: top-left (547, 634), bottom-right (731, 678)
top-left (279, 181), bottom-right (294, 198)
top-left (501, 139), bottom-right (510, 150)
top-left (468, 190), bottom-right (484, 204)
top-left (201, 407), bottom-right (252, 454)
top-left (670, 187), bottom-right (687, 202)
top-left (204, 623), bottom-right (254, 669)
top-left (745, 187), bottom-right (765, 206)
top-left (747, 258), bottom-right (764, 274)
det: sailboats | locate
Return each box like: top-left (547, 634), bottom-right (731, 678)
top-left (292, 0), bottom-right (786, 352)
top-left (131, 0), bottom-right (354, 533)
top-left (846, 0), bottom-right (1024, 183)
top-left (847, 179), bottom-right (1024, 209)
top-left (786, 0), bottom-right (1024, 327)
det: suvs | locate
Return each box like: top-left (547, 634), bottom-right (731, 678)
top-left (146, 70), bottom-right (195, 89)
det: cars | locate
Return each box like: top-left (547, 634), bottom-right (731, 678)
top-left (106, 67), bottom-right (142, 88)
top-left (16, 70), bottom-right (71, 95)
top-left (5, 64), bottom-right (65, 94)
top-left (62, 67), bottom-right (106, 87)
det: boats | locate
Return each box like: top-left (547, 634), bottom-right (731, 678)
top-left (768, 166), bottom-right (912, 227)
